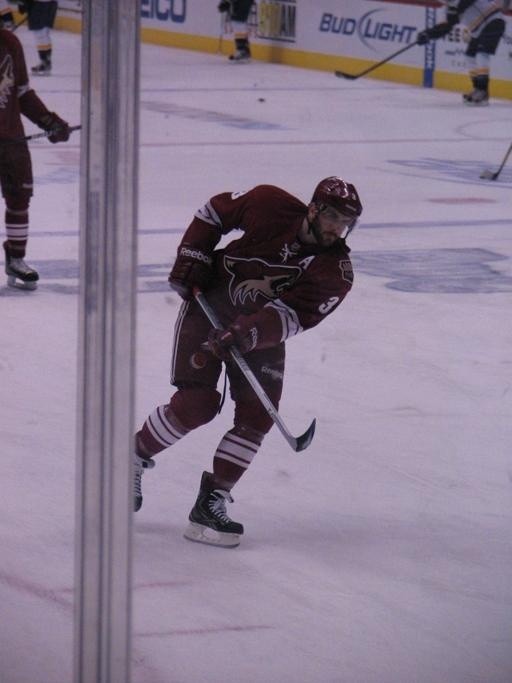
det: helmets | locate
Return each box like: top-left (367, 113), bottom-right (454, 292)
top-left (314, 175), bottom-right (363, 227)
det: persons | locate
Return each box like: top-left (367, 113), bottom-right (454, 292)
top-left (214, 1), bottom-right (257, 60)
top-left (132, 174), bottom-right (363, 537)
top-left (3, 0), bottom-right (59, 72)
top-left (1, 0), bottom-right (73, 284)
top-left (413, 0), bottom-right (512, 104)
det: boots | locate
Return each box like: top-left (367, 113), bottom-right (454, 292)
top-left (188, 471), bottom-right (243, 535)
top-left (134, 450), bottom-right (155, 512)
top-left (3, 241), bottom-right (39, 281)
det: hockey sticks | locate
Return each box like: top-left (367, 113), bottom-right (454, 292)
top-left (478, 143), bottom-right (512, 183)
top-left (191, 286), bottom-right (317, 453)
top-left (333, 38), bottom-right (419, 80)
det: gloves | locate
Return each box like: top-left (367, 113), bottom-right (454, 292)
top-left (209, 307), bottom-right (283, 359)
top-left (167, 245), bottom-right (215, 301)
top-left (417, 26), bottom-right (439, 45)
top-left (36, 111), bottom-right (70, 142)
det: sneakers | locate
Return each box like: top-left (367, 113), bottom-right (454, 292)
top-left (463, 89), bottom-right (488, 102)
top-left (31, 62), bottom-right (51, 71)
top-left (229, 47), bottom-right (250, 59)
